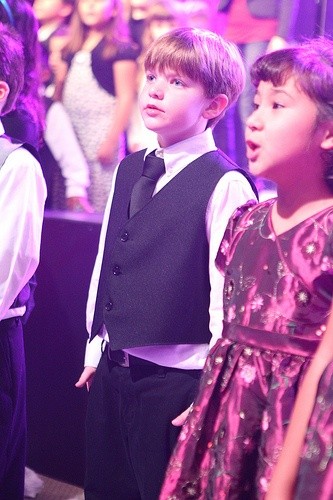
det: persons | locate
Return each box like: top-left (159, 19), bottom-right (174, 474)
top-left (0, 22), bottom-right (49, 500)
top-left (75, 26), bottom-right (256, 500)
top-left (0, 0), bottom-right (332, 218)
top-left (153, 33), bottom-right (333, 500)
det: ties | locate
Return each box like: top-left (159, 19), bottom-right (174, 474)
top-left (128, 149), bottom-right (166, 220)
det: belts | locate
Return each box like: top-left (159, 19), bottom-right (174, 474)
top-left (104, 342), bottom-right (203, 373)
